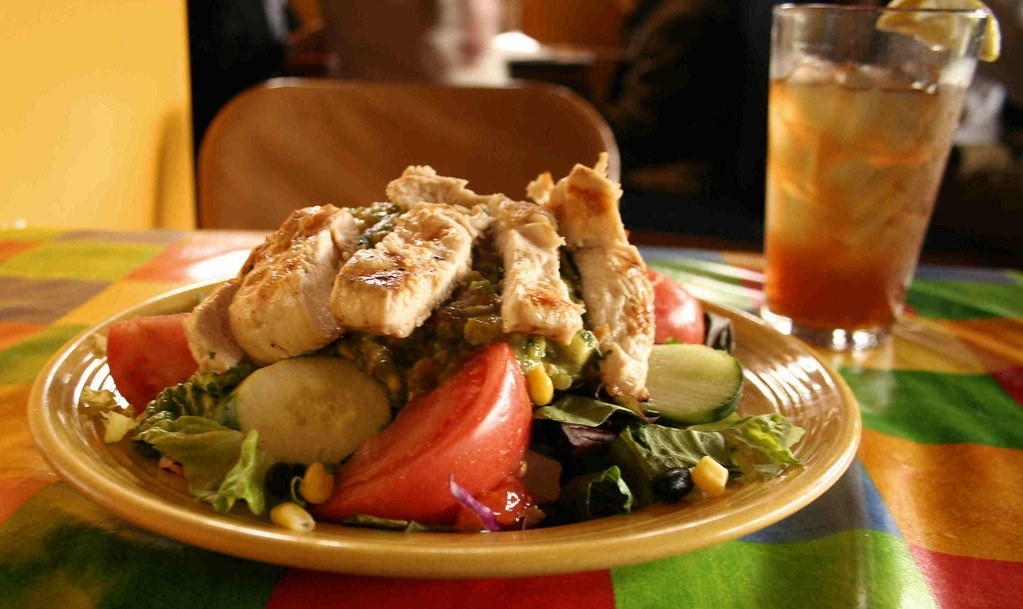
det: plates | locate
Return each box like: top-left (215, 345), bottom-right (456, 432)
top-left (24, 258), bottom-right (864, 579)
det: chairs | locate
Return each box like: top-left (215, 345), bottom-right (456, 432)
top-left (191, 79), bottom-right (622, 234)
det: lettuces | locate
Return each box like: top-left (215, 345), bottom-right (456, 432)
top-left (527, 330), bottom-right (804, 515)
top-left (80, 361), bottom-right (275, 517)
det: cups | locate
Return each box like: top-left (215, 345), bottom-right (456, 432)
top-left (757, 3), bottom-right (983, 353)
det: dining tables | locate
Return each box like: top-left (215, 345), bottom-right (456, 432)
top-left (0, 222), bottom-right (1021, 608)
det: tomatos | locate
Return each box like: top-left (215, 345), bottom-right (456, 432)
top-left (97, 311), bottom-right (203, 409)
top-left (322, 343), bottom-right (544, 534)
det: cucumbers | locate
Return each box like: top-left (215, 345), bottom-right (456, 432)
top-left (224, 354), bottom-right (388, 463)
